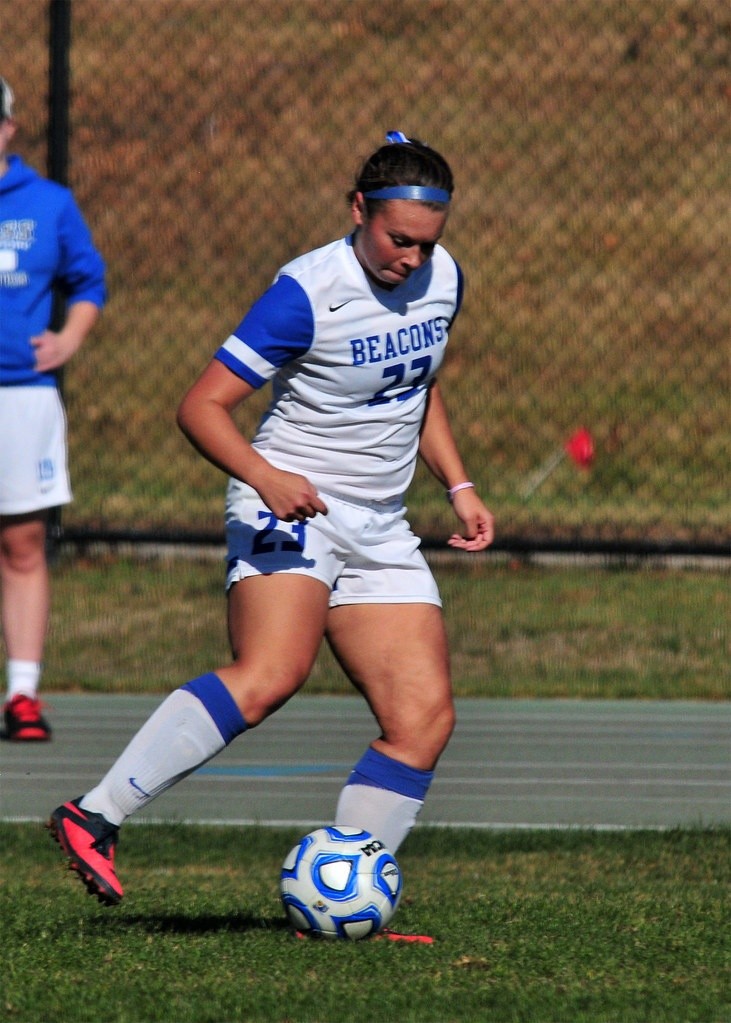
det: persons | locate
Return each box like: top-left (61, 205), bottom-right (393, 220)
top-left (45, 130), bottom-right (497, 945)
top-left (0, 79), bottom-right (107, 742)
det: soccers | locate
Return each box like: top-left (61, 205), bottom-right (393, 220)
top-left (277, 824), bottom-right (406, 942)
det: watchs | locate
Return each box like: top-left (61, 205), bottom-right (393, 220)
top-left (446, 481), bottom-right (475, 503)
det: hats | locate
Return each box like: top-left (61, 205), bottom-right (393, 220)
top-left (0, 77), bottom-right (15, 118)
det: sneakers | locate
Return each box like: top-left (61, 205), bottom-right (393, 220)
top-left (44, 795), bottom-right (123, 907)
top-left (0, 693), bottom-right (51, 740)
top-left (294, 924), bottom-right (433, 944)
